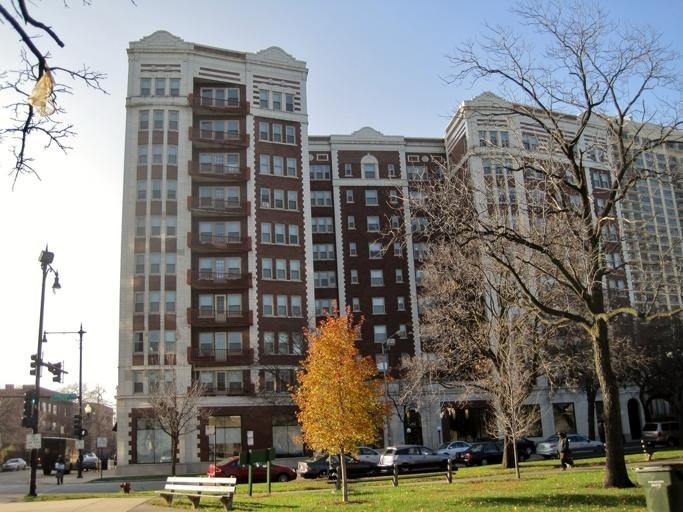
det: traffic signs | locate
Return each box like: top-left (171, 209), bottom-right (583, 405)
top-left (49, 393), bottom-right (76, 401)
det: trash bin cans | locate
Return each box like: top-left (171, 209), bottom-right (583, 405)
top-left (636, 464), bottom-right (683, 512)
top-left (108, 460), bottom-right (114, 472)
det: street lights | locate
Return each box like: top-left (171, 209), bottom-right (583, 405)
top-left (40, 323), bottom-right (87, 477)
top-left (382, 330), bottom-right (405, 448)
top-left (24, 242), bottom-right (62, 499)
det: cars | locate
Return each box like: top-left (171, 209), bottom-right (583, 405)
top-left (0, 457), bottom-right (27, 472)
top-left (454, 440), bottom-right (530, 467)
top-left (535, 434), bottom-right (605, 459)
top-left (206, 455), bottom-right (297, 483)
top-left (297, 452), bottom-right (381, 480)
top-left (160, 448), bottom-right (172, 463)
top-left (29, 435), bottom-right (117, 476)
top-left (481, 436), bottom-right (536, 457)
top-left (354, 446), bottom-right (383, 465)
top-left (434, 440), bottom-right (472, 462)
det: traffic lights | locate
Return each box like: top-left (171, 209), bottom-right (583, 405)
top-left (72, 413), bottom-right (79, 435)
top-left (29, 353), bottom-right (37, 376)
top-left (20, 416), bottom-right (33, 428)
top-left (51, 362), bottom-right (61, 383)
top-left (80, 428), bottom-right (86, 437)
top-left (22, 389), bottom-right (32, 417)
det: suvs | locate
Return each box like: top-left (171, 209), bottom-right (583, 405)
top-left (377, 445), bottom-right (454, 475)
top-left (641, 419), bottom-right (682, 448)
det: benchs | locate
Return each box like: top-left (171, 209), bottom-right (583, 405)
top-left (154, 475), bottom-right (237, 510)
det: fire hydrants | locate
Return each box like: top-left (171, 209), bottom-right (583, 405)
top-left (119, 481), bottom-right (130, 494)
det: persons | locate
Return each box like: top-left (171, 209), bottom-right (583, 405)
top-left (53, 454), bottom-right (65, 484)
top-left (556, 431), bottom-right (575, 472)
